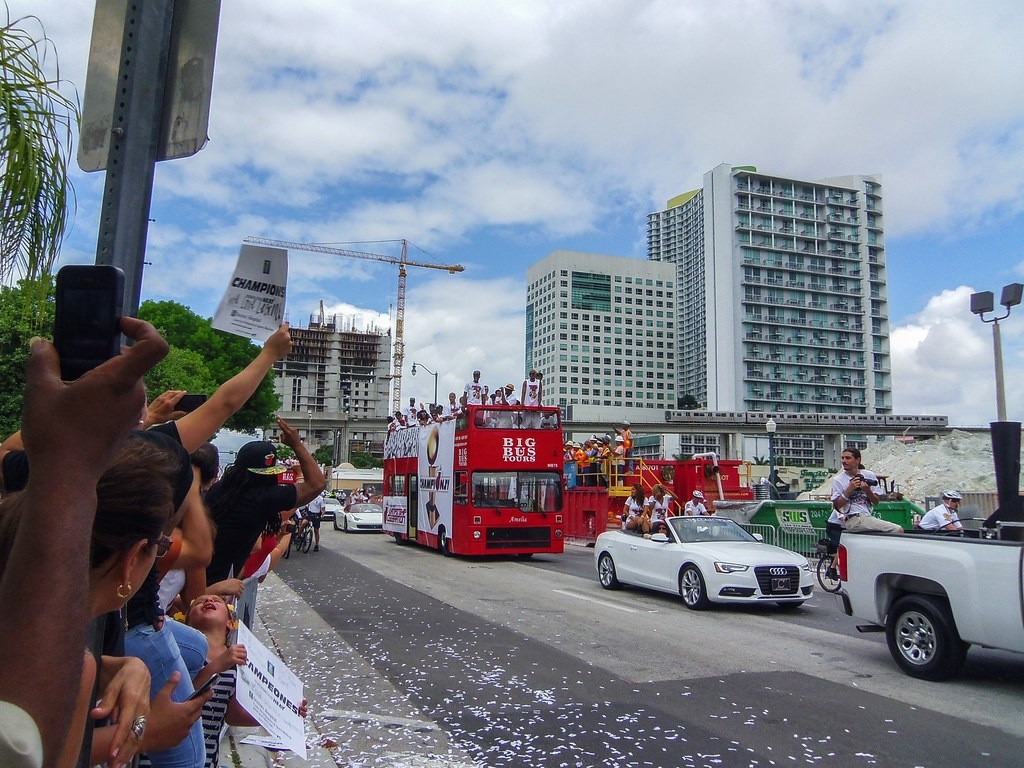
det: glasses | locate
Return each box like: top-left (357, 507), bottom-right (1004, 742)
top-left (949, 499), bottom-right (960, 503)
top-left (657, 526), bottom-right (667, 530)
top-left (151, 535), bottom-right (173, 558)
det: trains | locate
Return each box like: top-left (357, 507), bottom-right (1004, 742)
top-left (664, 410), bottom-right (948, 427)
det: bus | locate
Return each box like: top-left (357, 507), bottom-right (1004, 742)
top-left (382, 404), bottom-right (568, 559)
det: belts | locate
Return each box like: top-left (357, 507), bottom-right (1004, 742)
top-left (844, 513), bottom-right (860, 521)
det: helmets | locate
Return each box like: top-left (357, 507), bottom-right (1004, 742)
top-left (943, 491), bottom-right (963, 500)
top-left (693, 490), bottom-right (704, 498)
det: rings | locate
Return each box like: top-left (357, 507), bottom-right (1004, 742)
top-left (132, 716), bottom-right (146, 739)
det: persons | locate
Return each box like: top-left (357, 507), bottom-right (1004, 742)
top-left (237, 508), bottom-right (297, 583)
top-left (684, 490), bottom-right (710, 516)
top-left (462, 370), bottom-right (556, 429)
top-left (287, 495), bottom-right (326, 552)
top-left (831, 448), bottom-right (904, 534)
top-left (276, 455), bottom-right (300, 468)
top-left (623, 484), bottom-right (678, 543)
top-left (204, 419), bottom-right (327, 587)
top-left (563, 420), bottom-right (635, 487)
top-left (0, 315), bottom-right (293, 768)
top-left (322, 488), bottom-right (382, 513)
top-left (917, 490), bottom-right (964, 530)
top-left (825, 509), bottom-right (847, 581)
top-left (386, 392), bottom-right (467, 445)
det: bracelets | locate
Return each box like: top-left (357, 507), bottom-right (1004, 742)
top-left (641, 515), bottom-right (645, 518)
top-left (628, 515), bottom-right (632, 518)
top-left (654, 500), bottom-right (656, 503)
top-left (842, 493), bottom-right (848, 501)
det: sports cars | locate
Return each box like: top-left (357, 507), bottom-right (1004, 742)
top-left (333, 503), bottom-right (383, 533)
top-left (594, 515), bottom-right (815, 612)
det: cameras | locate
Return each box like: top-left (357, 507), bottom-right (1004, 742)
top-left (855, 473), bottom-right (878, 491)
top-left (286, 524), bottom-right (296, 532)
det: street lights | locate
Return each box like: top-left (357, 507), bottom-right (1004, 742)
top-left (766, 418), bottom-right (776, 500)
top-left (411, 362), bottom-right (438, 405)
top-left (971, 283), bottom-right (1024, 422)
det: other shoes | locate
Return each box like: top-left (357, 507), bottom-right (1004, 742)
top-left (313, 545), bottom-right (319, 551)
top-left (827, 565), bottom-right (838, 581)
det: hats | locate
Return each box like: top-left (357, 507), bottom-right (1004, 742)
top-left (614, 435), bottom-right (624, 442)
top-left (655, 522), bottom-right (671, 535)
top-left (235, 441), bottom-right (288, 475)
top-left (573, 443), bottom-right (581, 448)
top-left (473, 371), bottom-right (481, 375)
top-left (503, 384), bottom-right (515, 391)
top-left (602, 435), bottom-right (611, 442)
top-left (565, 440), bottom-right (573, 445)
top-left (622, 420), bottom-right (631, 426)
top-left (589, 435), bottom-right (597, 441)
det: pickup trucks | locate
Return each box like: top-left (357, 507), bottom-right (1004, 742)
top-left (839, 519), bottom-right (1024, 683)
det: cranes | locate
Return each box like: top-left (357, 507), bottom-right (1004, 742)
top-left (241, 235), bottom-right (466, 418)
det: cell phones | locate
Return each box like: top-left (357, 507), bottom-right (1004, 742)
top-left (174, 394), bottom-right (208, 412)
top-left (185, 673), bottom-right (222, 702)
top-left (53, 265), bottom-right (125, 381)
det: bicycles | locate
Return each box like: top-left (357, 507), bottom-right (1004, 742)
top-left (816, 538), bottom-right (842, 593)
top-left (283, 517), bottom-right (313, 559)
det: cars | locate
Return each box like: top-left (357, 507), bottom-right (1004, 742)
top-left (321, 497), bottom-right (342, 520)
